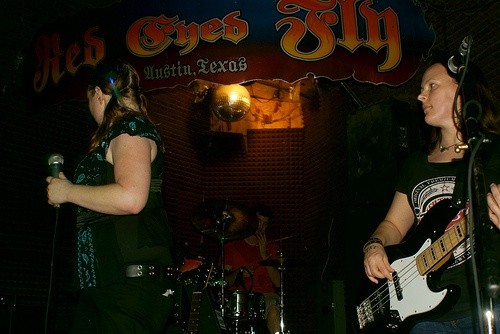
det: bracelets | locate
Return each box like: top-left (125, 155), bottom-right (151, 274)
top-left (362, 237), bottom-right (383, 252)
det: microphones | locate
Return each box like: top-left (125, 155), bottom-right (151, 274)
top-left (48, 153), bottom-right (66, 209)
top-left (447, 34), bottom-right (471, 74)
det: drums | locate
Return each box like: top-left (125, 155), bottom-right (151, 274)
top-left (166, 256), bottom-right (267, 334)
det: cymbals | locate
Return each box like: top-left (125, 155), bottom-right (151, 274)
top-left (258, 256), bottom-right (313, 266)
top-left (189, 198), bottom-right (259, 240)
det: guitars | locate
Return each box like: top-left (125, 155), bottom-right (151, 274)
top-left (186, 257), bottom-right (217, 334)
top-left (352, 212), bottom-right (474, 334)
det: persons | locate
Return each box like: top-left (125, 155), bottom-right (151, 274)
top-left (45, 51), bottom-right (173, 334)
top-left (363, 54), bottom-right (500, 334)
top-left (217, 207), bottom-right (281, 334)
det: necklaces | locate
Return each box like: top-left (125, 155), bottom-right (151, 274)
top-left (439, 139), bottom-right (463, 152)
